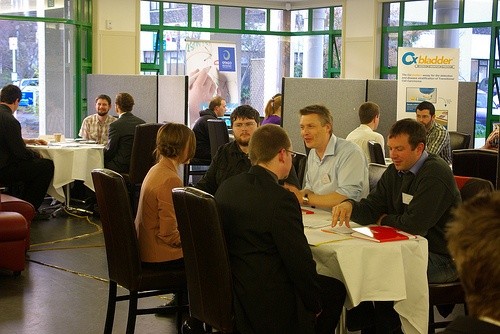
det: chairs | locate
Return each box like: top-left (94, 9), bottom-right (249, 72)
top-left (91, 118), bottom-right (500, 334)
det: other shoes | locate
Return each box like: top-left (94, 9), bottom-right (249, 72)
top-left (33, 210), bottom-right (50, 220)
top-left (184, 316), bottom-right (206, 334)
top-left (158, 295), bottom-right (189, 311)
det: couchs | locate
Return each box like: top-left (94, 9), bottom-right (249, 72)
top-left (0, 190), bottom-right (36, 277)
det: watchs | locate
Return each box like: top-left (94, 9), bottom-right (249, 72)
top-left (302, 194), bottom-right (309, 207)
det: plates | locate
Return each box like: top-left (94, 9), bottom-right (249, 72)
top-left (47, 139), bottom-right (104, 147)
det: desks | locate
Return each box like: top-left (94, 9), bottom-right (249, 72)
top-left (300, 205), bottom-right (429, 334)
top-left (24, 136), bottom-right (107, 220)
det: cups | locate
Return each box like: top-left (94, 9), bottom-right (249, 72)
top-left (54, 134), bottom-right (61, 142)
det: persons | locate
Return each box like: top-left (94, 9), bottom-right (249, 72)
top-left (331, 118), bottom-right (461, 334)
top-left (346, 101), bottom-right (385, 160)
top-left (71, 95), bottom-right (117, 216)
top-left (416, 101), bottom-right (452, 165)
top-left (188, 66), bottom-right (216, 129)
top-left (0, 84), bottom-right (54, 221)
top-left (135, 123), bottom-right (205, 334)
top-left (438, 191), bottom-right (500, 334)
top-left (192, 96), bottom-right (227, 160)
top-left (193, 105), bottom-right (301, 196)
top-left (213, 123), bottom-right (347, 334)
top-left (260, 96), bottom-right (282, 126)
top-left (482, 128), bottom-right (500, 151)
top-left (104, 92), bottom-right (146, 174)
top-left (281, 105), bottom-right (369, 212)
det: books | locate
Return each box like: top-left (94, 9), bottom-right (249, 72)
top-left (350, 226), bottom-right (409, 242)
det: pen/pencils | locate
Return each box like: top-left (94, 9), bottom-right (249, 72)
top-left (188, 66), bottom-right (211, 88)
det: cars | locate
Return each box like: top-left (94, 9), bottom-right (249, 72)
top-left (475, 88), bottom-right (500, 138)
top-left (19, 78), bottom-right (39, 93)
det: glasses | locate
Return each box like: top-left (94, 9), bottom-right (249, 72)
top-left (287, 149), bottom-right (296, 158)
